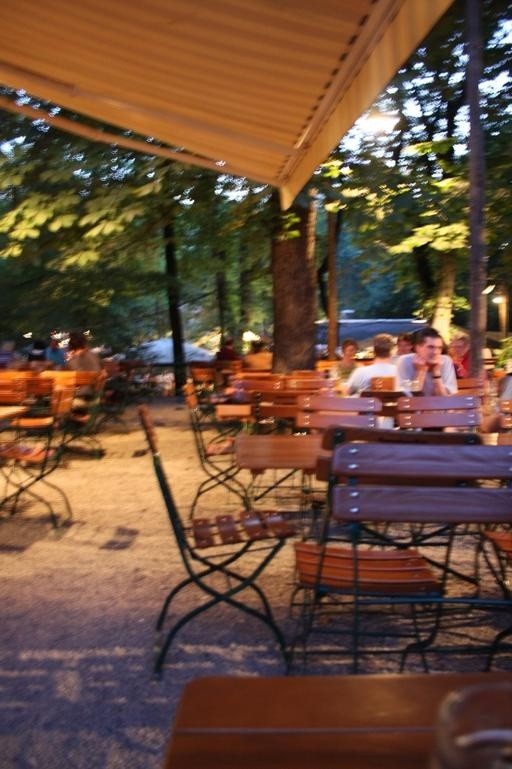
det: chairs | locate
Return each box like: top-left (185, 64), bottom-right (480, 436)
top-left (1, 367), bottom-right (132, 529)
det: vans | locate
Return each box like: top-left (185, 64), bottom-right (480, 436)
top-left (270, 320), bottom-right (431, 354)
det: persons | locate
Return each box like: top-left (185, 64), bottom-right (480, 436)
top-left (1, 332), bottom-right (100, 412)
top-left (217, 325), bottom-right (511, 450)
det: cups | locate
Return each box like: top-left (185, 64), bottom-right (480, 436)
top-left (425, 677), bottom-right (512, 769)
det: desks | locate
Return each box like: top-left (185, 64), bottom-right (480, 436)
top-left (164, 673), bottom-right (512, 769)
